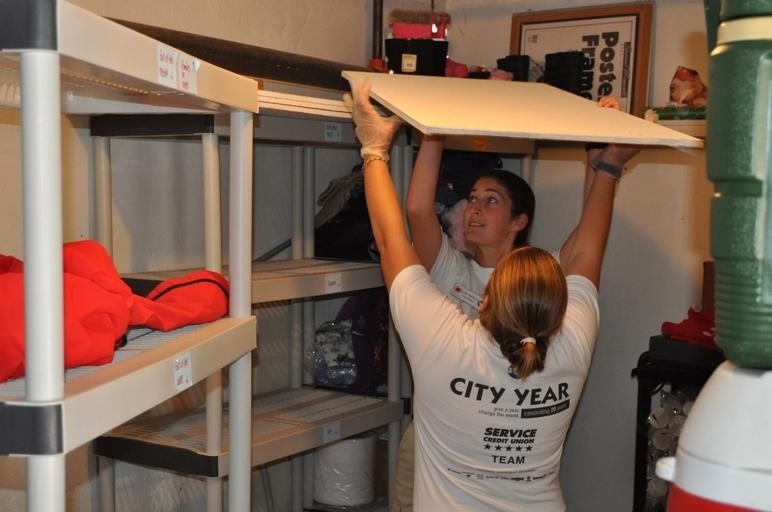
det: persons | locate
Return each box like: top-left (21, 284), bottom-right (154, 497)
top-left (341, 77), bottom-right (660, 512)
top-left (387, 74), bottom-right (621, 512)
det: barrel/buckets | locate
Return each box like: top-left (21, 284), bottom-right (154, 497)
top-left (709, 182), bottom-right (772, 370)
top-left (706, 16), bottom-right (772, 184)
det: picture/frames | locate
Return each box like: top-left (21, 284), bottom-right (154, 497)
top-left (509, 0), bottom-right (654, 120)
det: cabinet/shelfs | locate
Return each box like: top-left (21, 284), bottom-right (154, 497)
top-left (0, 2), bottom-right (261, 512)
top-left (88, 101), bottom-right (413, 512)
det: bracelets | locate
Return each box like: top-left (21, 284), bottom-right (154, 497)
top-left (595, 160), bottom-right (623, 182)
top-left (360, 155), bottom-right (389, 177)
top-left (584, 141), bottom-right (609, 153)
top-left (422, 135), bottom-right (448, 144)
